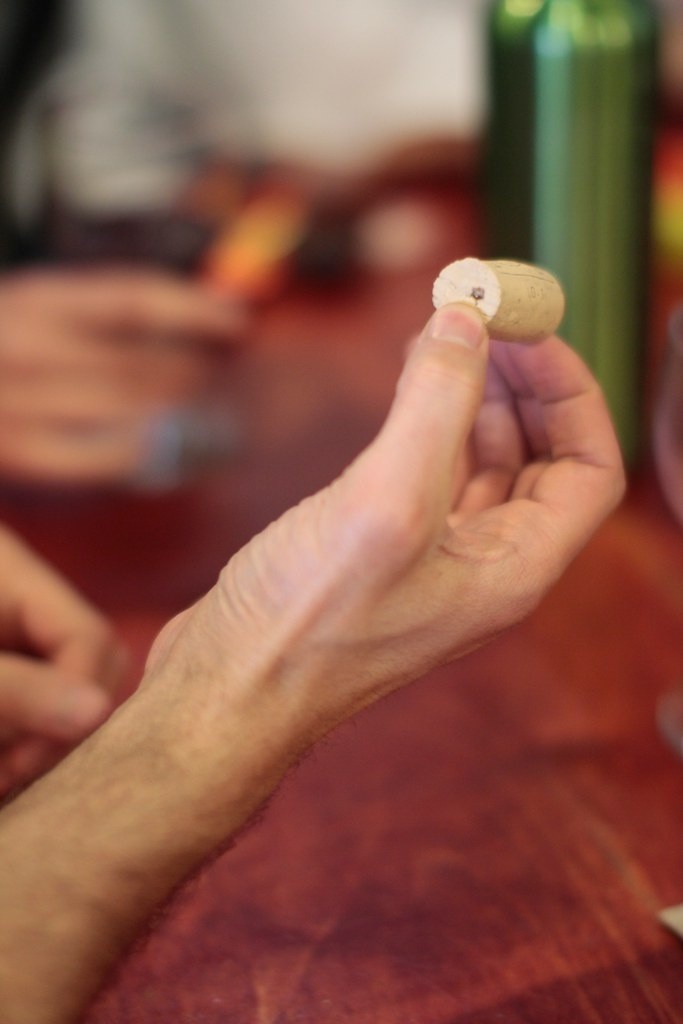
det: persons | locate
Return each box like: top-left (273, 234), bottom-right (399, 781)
top-left (2, 266), bottom-right (257, 491)
top-left (0, 303), bottom-right (626, 1022)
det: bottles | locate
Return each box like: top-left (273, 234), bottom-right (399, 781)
top-left (469, 0), bottom-right (683, 531)
